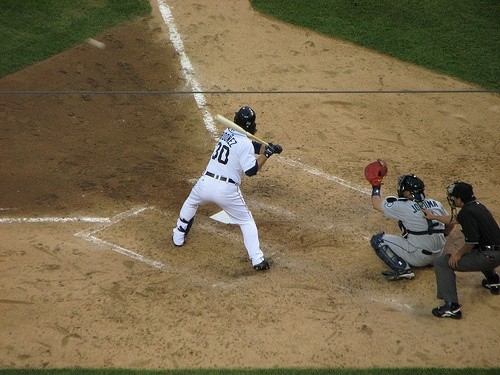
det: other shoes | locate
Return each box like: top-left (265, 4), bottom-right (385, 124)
top-left (382, 268), bottom-right (396, 276)
top-left (255, 260), bottom-right (270, 270)
top-left (386, 271), bottom-right (415, 281)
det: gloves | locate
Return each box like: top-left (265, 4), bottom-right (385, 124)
top-left (264, 142), bottom-right (275, 158)
top-left (274, 144), bottom-right (282, 154)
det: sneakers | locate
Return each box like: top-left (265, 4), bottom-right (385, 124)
top-left (431, 304), bottom-right (462, 319)
top-left (482, 279), bottom-right (500, 294)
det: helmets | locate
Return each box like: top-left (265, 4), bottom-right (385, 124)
top-left (446, 181), bottom-right (473, 198)
top-left (398, 174), bottom-right (425, 192)
top-left (235, 106), bottom-right (257, 127)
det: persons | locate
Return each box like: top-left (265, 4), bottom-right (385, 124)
top-left (421, 181), bottom-right (500, 319)
top-left (172, 106), bottom-right (283, 271)
top-left (364, 159), bottom-right (454, 282)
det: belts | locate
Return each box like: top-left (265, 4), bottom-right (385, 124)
top-left (475, 245), bottom-right (500, 251)
top-left (422, 249), bottom-right (433, 256)
top-left (206, 170), bottom-right (236, 184)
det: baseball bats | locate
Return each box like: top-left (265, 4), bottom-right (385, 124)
top-left (214, 113), bottom-right (270, 146)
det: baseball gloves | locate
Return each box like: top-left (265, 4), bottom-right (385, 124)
top-left (364, 157), bottom-right (389, 187)
